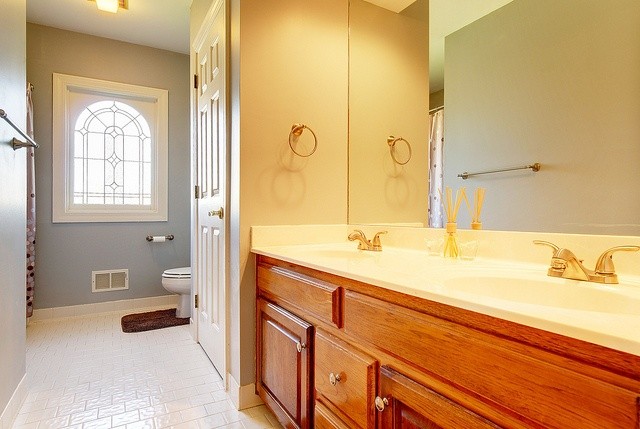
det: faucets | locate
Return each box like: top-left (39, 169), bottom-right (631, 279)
top-left (348, 229), bottom-right (388, 252)
top-left (532, 239), bottom-right (640, 284)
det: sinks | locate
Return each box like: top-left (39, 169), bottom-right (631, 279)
top-left (444, 277), bottom-right (639, 316)
top-left (297, 249), bottom-right (381, 269)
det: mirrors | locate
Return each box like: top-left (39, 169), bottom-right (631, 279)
top-left (347, 0), bottom-right (640, 241)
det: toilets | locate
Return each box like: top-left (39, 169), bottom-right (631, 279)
top-left (162, 267), bottom-right (191, 318)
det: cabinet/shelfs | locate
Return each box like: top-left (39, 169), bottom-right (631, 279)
top-left (348, 278), bottom-right (640, 428)
top-left (256, 253), bottom-right (346, 428)
top-left (311, 327), bottom-right (376, 428)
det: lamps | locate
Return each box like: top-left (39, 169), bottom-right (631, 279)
top-left (96, 0), bottom-right (121, 14)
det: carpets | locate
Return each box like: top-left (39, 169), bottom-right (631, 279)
top-left (121, 308), bottom-right (191, 332)
